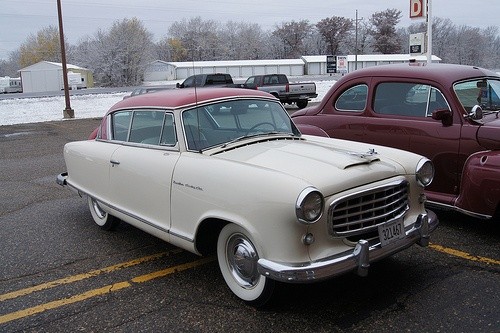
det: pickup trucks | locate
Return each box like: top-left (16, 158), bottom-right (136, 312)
top-left (174, 73), bottom-right (258, 90)
top-left (243, 74), bottom-right (318, 108)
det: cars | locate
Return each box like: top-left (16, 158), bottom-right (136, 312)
top-left (55, 87), bottom-right (441, 308)
top-left (123, 85), bottom-right (174, 101)
top-left (289, 63), bottom-right (500, 221)
